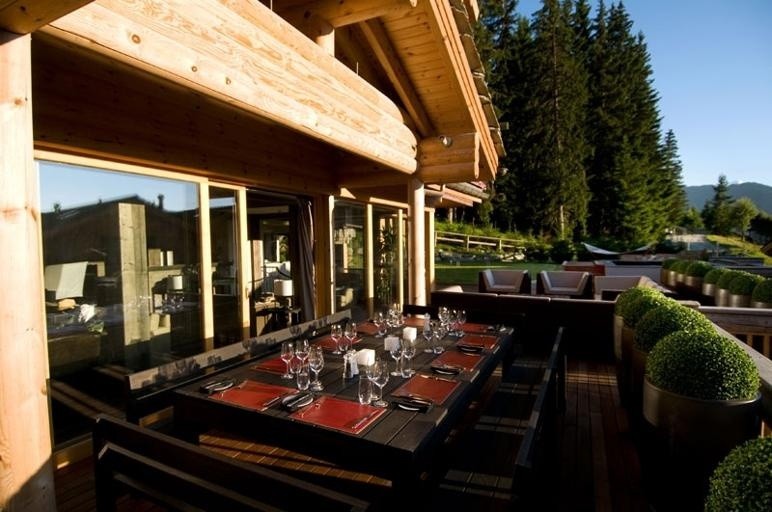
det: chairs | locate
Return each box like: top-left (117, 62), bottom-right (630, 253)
top-left (478, 269), bottom-right (531, 296)
top-left (537, 271), bottom-right (592, 300)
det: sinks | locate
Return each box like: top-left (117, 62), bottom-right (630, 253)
top-left (592, 274), bottom-right (678, 300)
top-left (431, 285), bottom-right (620, 363)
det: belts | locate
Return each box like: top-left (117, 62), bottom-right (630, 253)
top-left (423, 320), bottom-right (445, 352)
top-left (280, 338), bottom-right (325, 391)
top-left (438, 307), bottom-right (468, 338)
top-left (357, 356), bottom-right (390, 408)
top-left (330, 319), bottom-right (357, 353)
top-left (372, 305), bottom-right (405, 339)
top-left (389, 338), bottom-right (416, 378)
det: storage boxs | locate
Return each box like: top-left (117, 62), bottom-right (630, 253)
top-left (427, 326), bottom-right (568, 512)
top-left (124, 307), bottom-right (353, 434)
top-left (92, 413), bottom-right (372, 512)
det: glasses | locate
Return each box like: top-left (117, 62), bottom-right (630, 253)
top-left (274, 279), bottom-right (295, 328)
top-left (168, 275), bottom-right (183, 309)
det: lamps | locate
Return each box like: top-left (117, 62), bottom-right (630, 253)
top-left (168, 275), bottom-right (183, 309)
top-left (537, 271), bottom-right (592, 300)
top-left (274, 279), bottom-right (295, 328)
top-left (478, 269), bottom-right (531, 296)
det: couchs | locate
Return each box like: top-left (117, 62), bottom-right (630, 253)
top-left (592, 274), bottom-right (678, 300)
top-left (431, 285), bottom-right (620, 363)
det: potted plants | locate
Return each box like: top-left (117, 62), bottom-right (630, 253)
top-left (615, 286), bottom-right (681, 360)
top-left (629, 304), bottom-right (719, 387)
top-left (726, 274), bottom-right (766, 307)
top-left (661, 260), bottom-right (732, 298)
top-left (705, 436), bottom-right (770, 510)
top-left (643, 330), bottom-right (761, 436)
top-left (751, 277), bottom-right (771, 308)
top-left (715, 271), bottom-right (749, 307)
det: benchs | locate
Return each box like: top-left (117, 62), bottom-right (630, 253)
top-left (92, 413), bottom-right (372, 512)
top-left (427, 326), bottom-right (568, 512)
top-left (124, 307), bottom-right (353, 434)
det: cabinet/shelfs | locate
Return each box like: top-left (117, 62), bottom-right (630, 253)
top-left (423, 313), bottom-right (431, 331)
top-left (342, 349), bottom-right (359, 378)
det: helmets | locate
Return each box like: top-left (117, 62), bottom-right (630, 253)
top-left (460, 344), bottom-right (479, 352)
top-left (281, 395), bottom-right (313, 407)
top-left (434, 364), bottom-right (459, 374)
top-left (202, 380), bottom-right (234, 390)
top-left (400, 393), bottom-right (424, 411)
